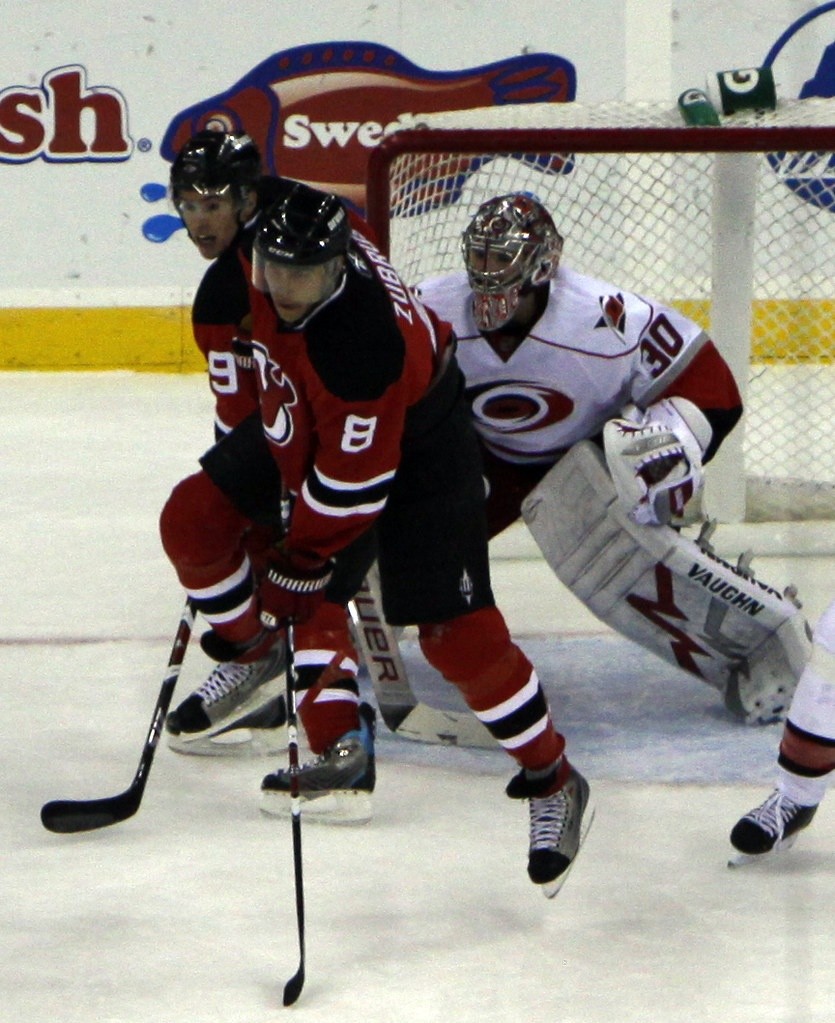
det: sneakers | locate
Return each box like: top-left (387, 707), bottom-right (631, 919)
top-left (727, 789), bottom-right (819, 866)
top-left (255, 703), bottom-right (377, 824)
top-left (165, 643), bottom-right (298, 755)
top-left (528, 756), bottom-right (597, 898)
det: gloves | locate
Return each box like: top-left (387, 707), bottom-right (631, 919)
top-left (255, 552), bottom-right (336, 629)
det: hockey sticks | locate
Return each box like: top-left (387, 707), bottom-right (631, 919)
top-left (39, 596), bottom-right (198, 835)
top-left (347, 564), bottom-right (505, 749)
top-left (280, 498), bottom-right (307, 1007)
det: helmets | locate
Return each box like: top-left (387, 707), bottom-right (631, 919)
top-left (170, 127), bottom-right (267, 205)
top-left (254, 183), bottom-right (350, 333)
top-left (459, 195), bottom-right (567, 331)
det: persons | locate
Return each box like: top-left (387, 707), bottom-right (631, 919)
top-left (157, 130), bottom-right (835, 884)
